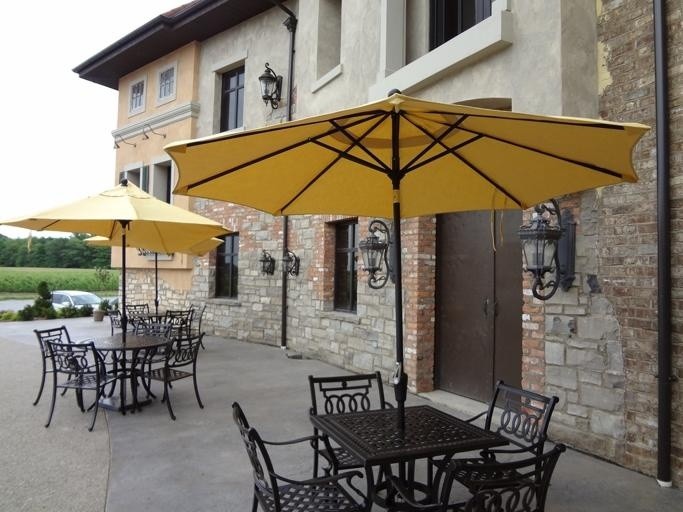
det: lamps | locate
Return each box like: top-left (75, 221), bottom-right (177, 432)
top-left (359, 219), bottom-right (396, 289)
top-left (113, 134), bottom-right (136, 149)
top-left (281, 248), bottom-right (300, 277)
top-left (259, 249), bottom-right (274, 275)
top-left (141, 123), bottom-right (166, 141)
top-left (517, 197), bottom-right (576, 301)
top-left (258, 62), bottom-right (283, 109)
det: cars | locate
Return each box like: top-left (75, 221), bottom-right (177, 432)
top-left (49, 290), bottom-right (101, 314)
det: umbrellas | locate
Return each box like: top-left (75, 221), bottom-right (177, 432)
top-left (83, 234), bottom-right (226, 314)
top-left (163, 88), bottom-right (653, 477)
top-left (0, 177), bottom-right (236, 400)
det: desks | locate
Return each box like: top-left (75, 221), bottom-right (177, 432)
top-left (308, 404), bottom-right (509, 512)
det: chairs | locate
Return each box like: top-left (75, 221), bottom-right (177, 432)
top-left (32, 304), bottom-right (208, 432)
top-left (388, 443), bottom-right (567, 512)
top-left (308, 371), bottom-right (416, 511)
top-left (427, 379), bottom-right (560, 512)
top-left (230, 401), bottom-right (368, 512)
